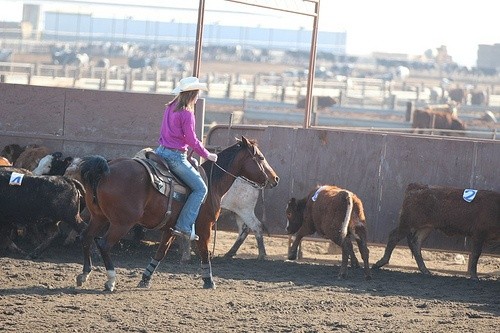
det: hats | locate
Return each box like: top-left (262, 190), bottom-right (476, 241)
top-left (172, 77), bottom-right (207, 93)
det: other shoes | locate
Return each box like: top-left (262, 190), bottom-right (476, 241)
top-left (175, 228), bottom-right (200, 240)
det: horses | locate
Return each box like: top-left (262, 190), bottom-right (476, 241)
top-left (76, 135), bottom-right (281, 292)
top-left (372, 182), bottom-right (500, 282)
top-left (284, 184), bottom-right (370, 279)
top-left (0, 143), bottom-right (145, 259)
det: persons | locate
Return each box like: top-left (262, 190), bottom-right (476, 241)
top-left (155, 76), bottom-right (218, 241)
